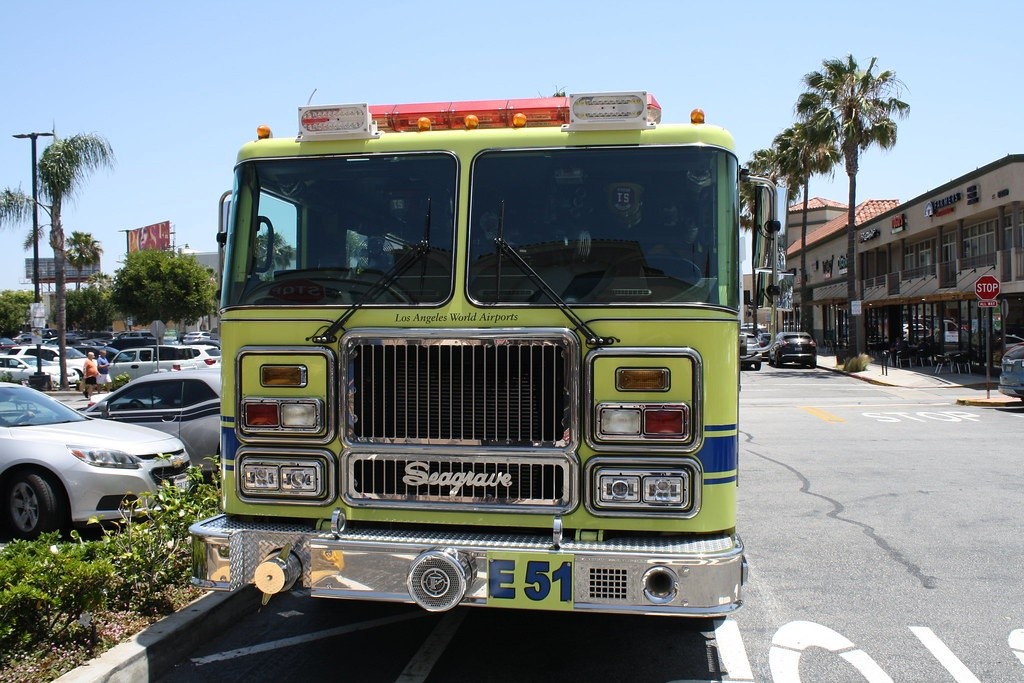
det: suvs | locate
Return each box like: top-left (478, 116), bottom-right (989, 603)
top-left (768, 331), bottom-right (817, 369)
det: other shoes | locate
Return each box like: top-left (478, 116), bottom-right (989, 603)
top-left (83, 391), bottom-right (89, 398)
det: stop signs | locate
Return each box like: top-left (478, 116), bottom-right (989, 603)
top-left (975, 275), bottom-right (1001, 300)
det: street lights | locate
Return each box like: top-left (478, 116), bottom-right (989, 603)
top-left (11, 133), bottom-right (55, 368)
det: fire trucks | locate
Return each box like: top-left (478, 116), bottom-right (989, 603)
top-left (187, 89), bottom-right (795, 639)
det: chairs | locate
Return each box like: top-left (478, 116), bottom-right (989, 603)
top-left (867, 341), bottom-right (971, 374)
top-left (371, 240), bottom-right (712, 295)
top-left (824, 338), bottom-right (850, 354)
top-left (154, 392), bottom-right (176, 407)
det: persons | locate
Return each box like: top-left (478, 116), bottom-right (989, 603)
top-left (127, 316), bottom-right (133, 331)
top-left (79, 349), bottom-right (112, 400)
top-left (890, 337), bottom-right (930, 367)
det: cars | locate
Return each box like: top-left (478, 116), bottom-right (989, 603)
top-left (71, 364), bottom-right (222, 477)
top-left (998, 340), bottom-right (1024, 402)
top-left (903, 324), bottom-right (927, 342)
top-left (0, 327), bottom-right (222, 391)
top-left (944, 319), bottom-right (958, 343)
top-left (740, 322), bottom-right (772, 371)
top-left (0, 382), bottom-right (197, 543)
